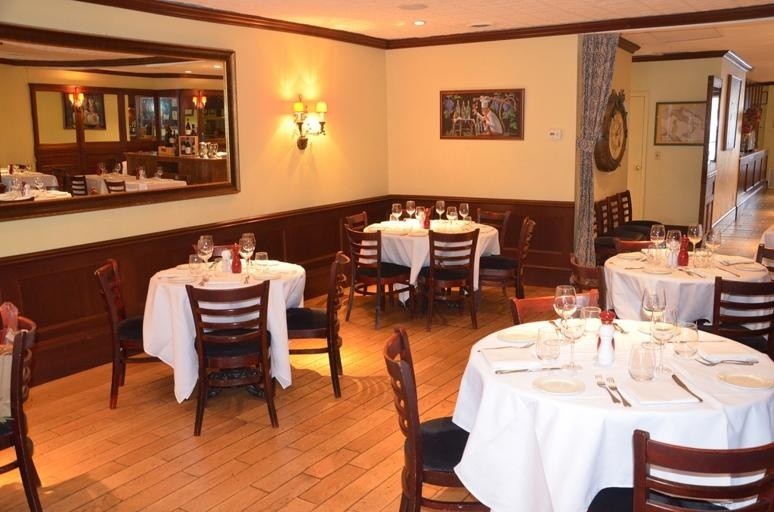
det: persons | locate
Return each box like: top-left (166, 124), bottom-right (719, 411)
top-left (472, 100), bottom-right (503, 134)
top-left (84, 98), bottom-right (101, 131)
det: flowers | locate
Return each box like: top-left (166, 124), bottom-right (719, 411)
top-left (742, 103), bottom-right (763, 133)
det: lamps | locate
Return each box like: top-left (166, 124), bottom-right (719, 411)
top-left (67, 87), bottom-right (84, 112)
top-left (292, 95), bottom-right (327, 150)
top-left (191, 88), bottom-right (208, 111)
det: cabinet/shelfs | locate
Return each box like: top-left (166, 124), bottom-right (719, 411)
top-left (121, 152), bottom-right (228, 187)
top-left (180, 89), bottom-right (226, 142)
top-left (736, 148), bottom-right (768, 219)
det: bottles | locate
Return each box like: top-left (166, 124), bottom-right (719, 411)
top-left (181, 118), bottom-right (197, 157)
top-left (231, 244), bottom-right (244, 274)
top-left (220, 250), bottom-right (230, 274)
top-left (677, 233), bottom-right (690, 266)
top-left (422, 206), bottom-right (431, 231)
top-left (594, 309), bottom-right (616, 366)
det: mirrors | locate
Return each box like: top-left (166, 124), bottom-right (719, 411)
top-left (0, 20), bottom-right (242, 222)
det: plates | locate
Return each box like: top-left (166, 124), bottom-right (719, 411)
top-left (500, 333), bottom-right (534, 344)
top-left (644, 268), bottom-right (672, 275)
top-left (734, 264), bottom-right (765, 273)
top-left (531, 375), bottom-right (584, 396)
top-left (714, 369), bottom-right (774, 395)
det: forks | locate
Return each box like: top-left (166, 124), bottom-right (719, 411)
top-left (595, 374), bottom-right (632, 408)
top-left (693, 352), bottom-right (759, 368)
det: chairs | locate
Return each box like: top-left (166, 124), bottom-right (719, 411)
top-left (0, 156), bottom-right (187, 208)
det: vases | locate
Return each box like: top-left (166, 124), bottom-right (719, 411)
top-left (741, 130), bottom-right (756, 153)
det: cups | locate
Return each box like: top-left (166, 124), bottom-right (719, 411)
top-left (626, 341), bottom-right (656, 381)
top-left (535, 325), bottom-right (561, 360)
top-left (672, 322), bottom-right (699, 358)
top-left (189, 254), bottom-right (205, 276)
top-left (580, 306), bottom-right (601, 337)
top-left (254, 251), bottom-right (269, 273)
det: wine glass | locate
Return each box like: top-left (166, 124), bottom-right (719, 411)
top-left (554, 281), bottom-right (677, 375)
top-left (647, 223), bottom-right (721, 274)
top-left (183, 232), bottom-right (269, 279)
top-left (96, 160), bottom-right (164, 183)
top-left (3, 162), bottom-right (44, 199)
top-left (386, 198), bottom-right (472, 230)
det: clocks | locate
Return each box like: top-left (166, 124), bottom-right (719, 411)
top-left (593, 88), bottom-right (628, 172)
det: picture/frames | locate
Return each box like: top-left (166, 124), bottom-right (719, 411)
top-left (439, 88), bottom-right (526, 141)
top-left (653, 101), bottom-right (707, 146)
top-left (761, 90), bottom-right (768, 105)
top-left (61, 91), bottom-right (107, 130)
top-left (723, 74), bottom-right (742, 151)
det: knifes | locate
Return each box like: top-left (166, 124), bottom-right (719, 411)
top-left (670, 372), bottom-right (704, 405)
top-left (491, 365), bottom-right (565, 377)
